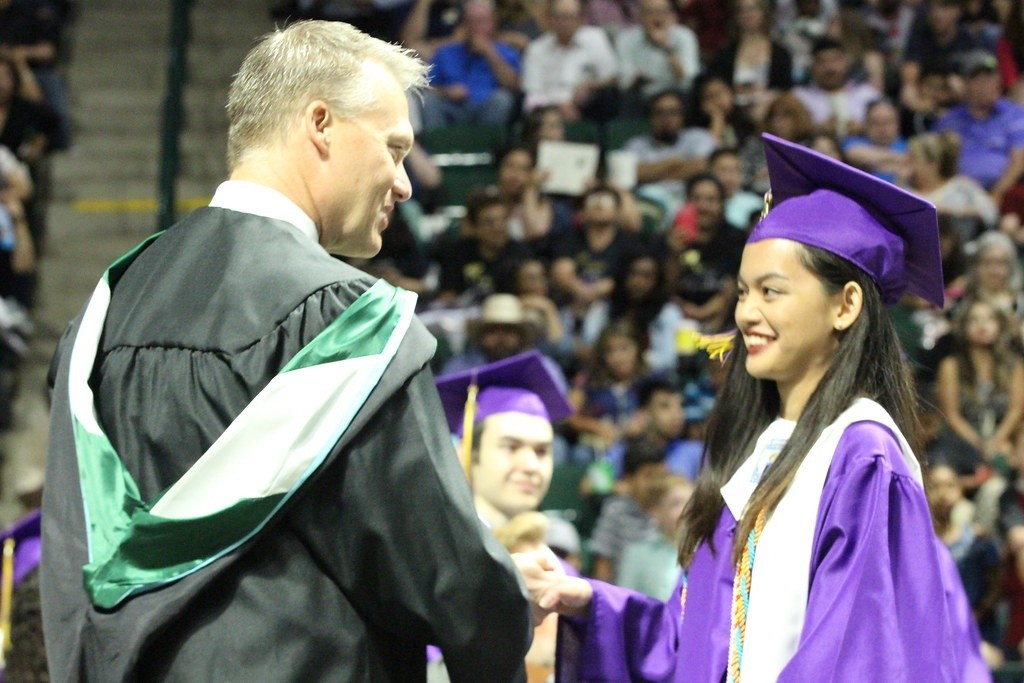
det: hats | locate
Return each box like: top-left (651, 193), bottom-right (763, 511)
top-left (468, 294), bottom-right (539, 327)
top-left (967, 51), bottom-right (997, 75)
top-left (691, 132), bottom-right (945, 363)
top-left (0, 508), bottom-right (41, 667)
top-left (921, 427), bottom-right (981, 476)
top-left (435, 351), bottom-right (575, 488)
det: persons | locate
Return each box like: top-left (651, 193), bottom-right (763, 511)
top-left (36, 14), bottom-right (561, 682)
top-left (271, 0), bottom-right (1024, 683)
top-left (1, 0), bottom-right (82, 423)
